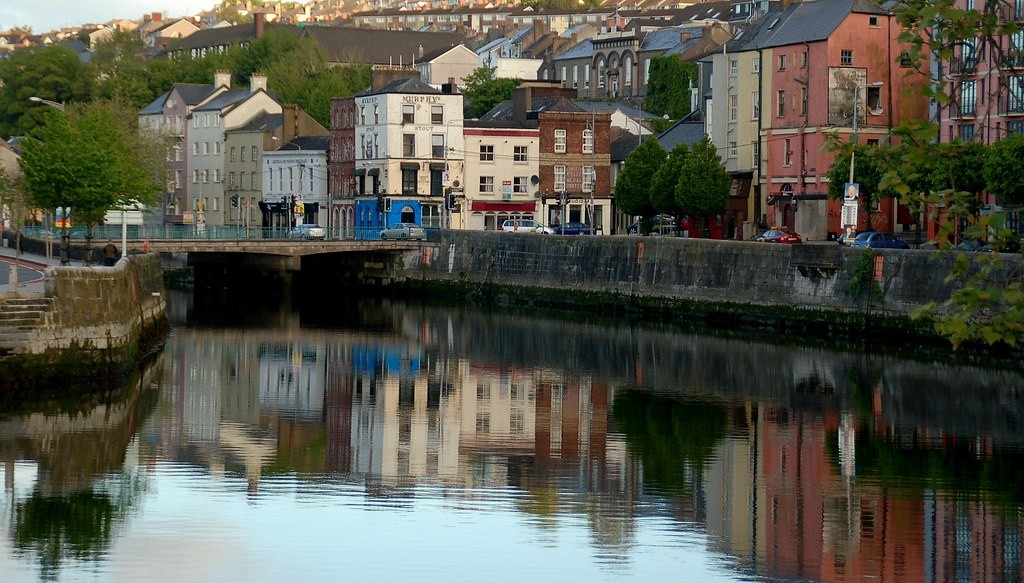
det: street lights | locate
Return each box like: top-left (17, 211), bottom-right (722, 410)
top-left (272, 137), bottom-right (301, 193)
top-left (30, 96), bottom-right (127, 259)
top-left (443, 118), bottom-right (479, 228)
top-left (846, 81), bottom-right (883, 245)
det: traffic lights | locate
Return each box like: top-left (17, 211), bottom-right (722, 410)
top-left (292, 194), bottom-right (297, 208)
top-left (556, 193), bottom-right (561, 205)
top-left (542, 194), bottom-right (546, 204)
top-left (565, 192), bottom-right (571, 204)
top-left (448, 194), bottom-right (454, 209)
top-left (379, 200), bottom-right (384, 211)
top-left (232, 196), bottom-right (237, 207)
top-left (386, 198), bottom-right (390, 211)
top-left (280, 196), bottom-right (286, 208)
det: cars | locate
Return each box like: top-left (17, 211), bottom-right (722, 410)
top-left (40, 230), bottom-right (56, 239)
top-left (627, 214), bottom-right (678, 236)
top-left (756, 229), bottom-right (802, 244)
top-left (554, 222), bottom-right (596, 235)
top-left (836, 231), bottom-right (859, 244)
top-left (380, 223), bottom-right (425, 240)
top-left (55, 230), bottom-right (70, 239)
top-left (851, 232), bottom-right (910, 249)
top-left (289, 224), bottom-right (326, 241)
top-left (70, 231), bottom-right (88, 238)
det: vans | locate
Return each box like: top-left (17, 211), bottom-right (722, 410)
top-left (502, 220), bottom-right (556, 234)
